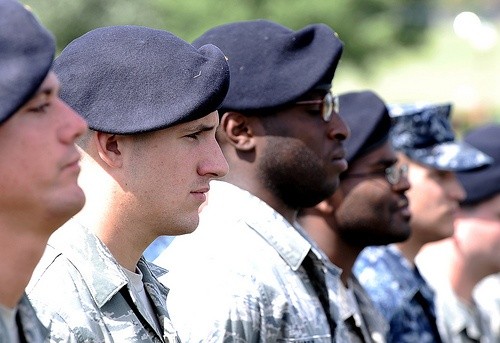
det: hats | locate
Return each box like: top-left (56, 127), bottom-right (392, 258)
top-left (189, 20), bottom-right (344, 116)
top-left (331, 90), bottom-right (393, 173)
top-left (0, 0), bottom-right (56, 126)
top-left (51, 25), bottom-right (230, 136)
top-left (381, 101), bottom-right (495, 174)
top-left (453, 122), bottom-right (500, 206)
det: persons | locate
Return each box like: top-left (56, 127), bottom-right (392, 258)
top-left (159, 21), bottom-right (355, 343)
top-left (25, 26), bottom-right (230, 343)
top-left (291, 90), bottom-right (412, 343)
top-left (419, 124), bottom-right (500, 343)
top-left (351, 103), bottom-right (494, 343)
top-left (1, 0), bottom-right (88, 343)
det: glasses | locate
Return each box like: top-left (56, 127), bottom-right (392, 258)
top-left (284, 92), bottom-right (340, 123)
top-left (339, 161), bottom-right (410, 184)
